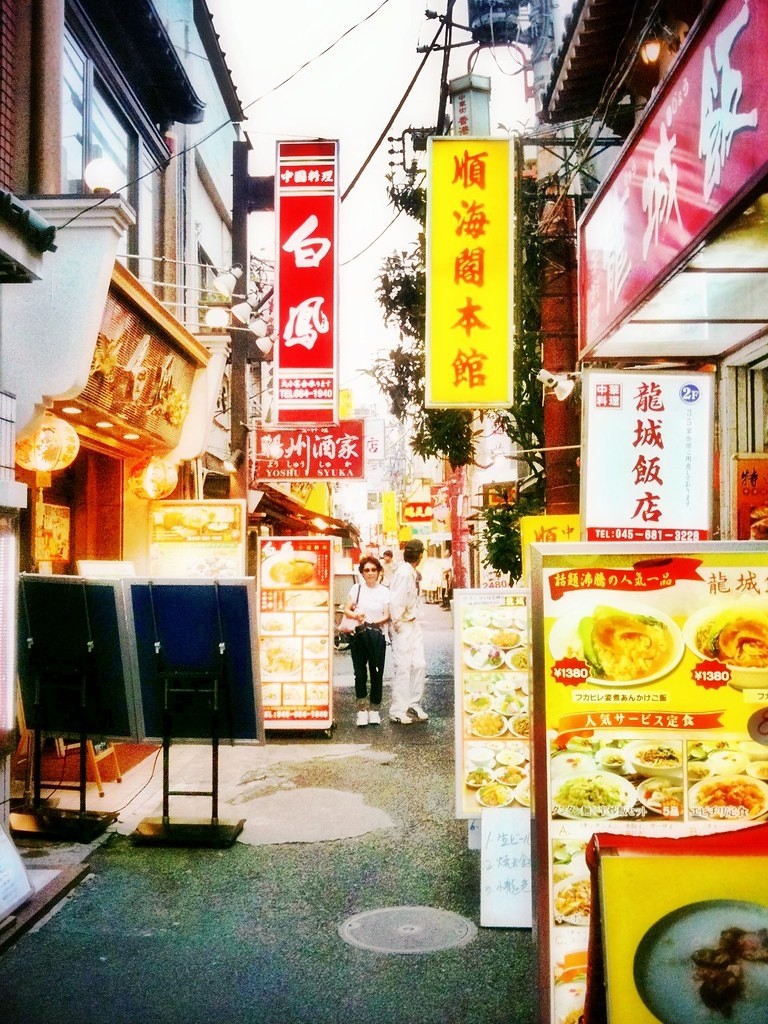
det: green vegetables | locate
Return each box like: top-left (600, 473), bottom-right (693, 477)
top-left (579, 607), bottom-right (666, 677)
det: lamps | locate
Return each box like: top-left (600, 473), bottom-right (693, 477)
top-left (529, 365), bottom-right (584, 404)
top-left (83, 158), bottom-right (125, 197)
top-left (640, 20), bottom-right (681, 67)
top-left (117, 252), bottom-right (281, 353)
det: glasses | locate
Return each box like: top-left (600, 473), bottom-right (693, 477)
top-left (363, 568), bottom-right (379, 572)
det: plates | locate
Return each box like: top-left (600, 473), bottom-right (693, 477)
top-left (548, 601), bottom-right (683, 686)
top-left (683, 605), bottom-right (768, 672)
top-left (546, 743), bottom-right (767, 1023)
top-left (261, 551), bottom-right (316, 588)
top-left (466, 606), bottom-right (532, 809)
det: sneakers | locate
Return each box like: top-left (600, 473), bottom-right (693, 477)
top-left (409, 704), bottom-right (428, 720)
top-left (356, 709), bottom-right (369, 726)
top-left (368, 709), bottom-right (381, 724)
top-left (390, 711), bottom-right (413, 724)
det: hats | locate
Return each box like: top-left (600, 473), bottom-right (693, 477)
top-left (405, 540), bottom-right (428, 553)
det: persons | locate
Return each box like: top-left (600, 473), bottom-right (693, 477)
top-left (361, 549), bottom-right (422, 597)
top-left (387, 539), bottom-right (430, 724)
top-left (440, 549), bottom-right (453, 611)
top-left (343, 556), bottom-right (390, 725)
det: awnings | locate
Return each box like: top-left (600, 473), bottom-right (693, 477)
top-left (254, 482), bottom-right (360, 541)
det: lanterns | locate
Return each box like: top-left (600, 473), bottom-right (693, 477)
top-left (129, 456), bottom-right (178, 500)
top-left (16, 417), bottom-right (79, 487)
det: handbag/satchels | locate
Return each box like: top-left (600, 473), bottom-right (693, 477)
top-left (338, 585), bottom-right (361, 633)
